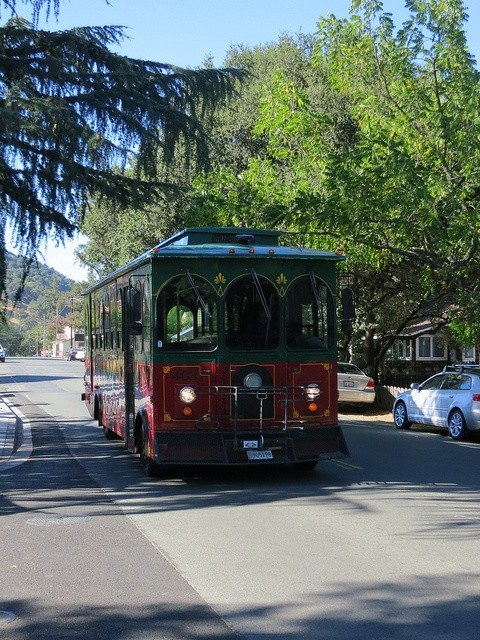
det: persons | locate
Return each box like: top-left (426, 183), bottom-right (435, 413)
top-left (241, 300), bottom-right (289, 348)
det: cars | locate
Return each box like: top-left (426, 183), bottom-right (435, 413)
top-left (336, 361), bottom-right (376, 412)
top-left (0, 344), bottom-right (7, 362)
top-left (64, 348), bottom-right (77, 361)
top-left (392, 364), bottom-right (480, 440)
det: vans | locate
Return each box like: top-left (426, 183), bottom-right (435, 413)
top-left (76, 347), bottom-right (85, 362)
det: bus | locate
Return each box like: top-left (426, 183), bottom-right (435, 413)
top-left (81, 227), bottom-right (349, 475)
